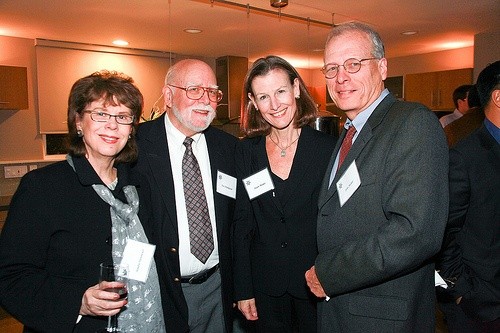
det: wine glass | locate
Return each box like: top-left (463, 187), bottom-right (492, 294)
top-left (99, 262), bottom-right (127, 333)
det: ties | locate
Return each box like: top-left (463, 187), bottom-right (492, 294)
top-left (181, 137), bottom-right (215, 264)
top-left (337, 125), bottom-right (357, 170)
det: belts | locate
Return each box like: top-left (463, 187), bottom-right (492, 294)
top-left (180, 262), bottom-right (220, 285)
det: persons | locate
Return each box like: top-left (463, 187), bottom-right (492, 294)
top-left (230, 56), bottom-right (338, 333)
top-left (435, 60), bottom-right (500, 333)
top-left (0, 70), bottom-right (190, 333)
top-left (126, 58), bottom-right (240, 333)
top-left (305, 20), bottom-right (450, 333)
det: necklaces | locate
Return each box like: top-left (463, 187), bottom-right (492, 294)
top-left (269, 134), bottom-right (299, 157)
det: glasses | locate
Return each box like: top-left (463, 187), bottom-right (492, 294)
top-left (81, 110), bottom-right (135, 125)
top-left (167, 84), bottom-right (224, 103)
top-left (319, 57), bottom-right (381, 79)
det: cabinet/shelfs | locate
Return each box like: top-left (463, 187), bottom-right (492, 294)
top-left (404, 68), bottom-right (474, 112)
top-left (0, 65), bottom-right (29, 110)
